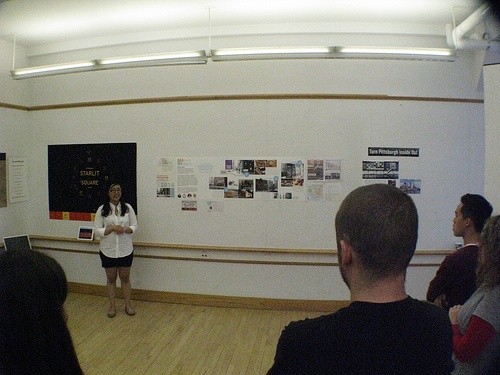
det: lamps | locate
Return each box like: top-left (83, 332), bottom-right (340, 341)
top-left (10, 8), bottom-right (457, 80)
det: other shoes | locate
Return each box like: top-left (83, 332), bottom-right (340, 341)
top-left (125, 307), bottom-right (135, 315)
top-left (108, 309), bottom-right (116, 318)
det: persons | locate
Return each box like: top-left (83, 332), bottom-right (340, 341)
top-left (0, 249), bottom-right (84, 374)
top-left (267, 184), bottom-right (457, 375)
top-left (427, 194), bottom-right (493, 313)
top-left (449, 214), bottom-right (500, 375)
top-left (95, 179), bottom-right (139, 318)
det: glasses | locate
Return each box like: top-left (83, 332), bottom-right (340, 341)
top-left (109, 189), bottom-right (122, 193)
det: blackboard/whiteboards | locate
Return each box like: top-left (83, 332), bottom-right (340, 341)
top-left (0, 92), bottom-right (488, 258)
